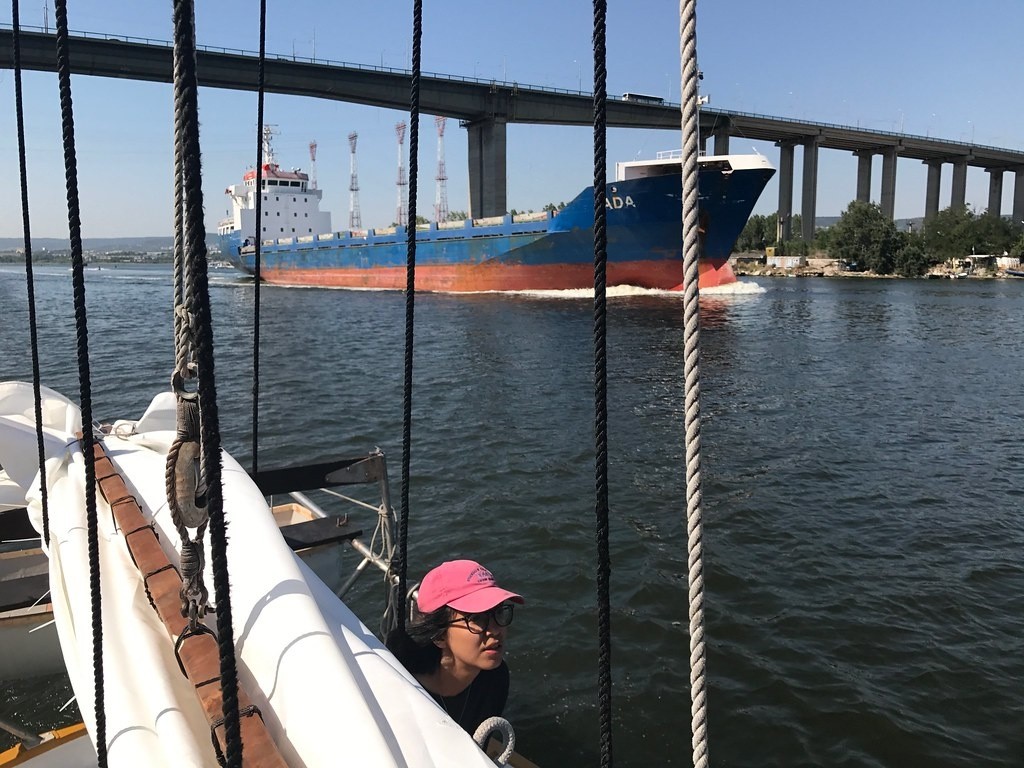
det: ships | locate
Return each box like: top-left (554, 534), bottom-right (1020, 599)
top-left (214, 121), bottom-right (774, 291)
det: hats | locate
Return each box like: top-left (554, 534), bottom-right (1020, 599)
top-left (417, 559), bottom-right (526, 613)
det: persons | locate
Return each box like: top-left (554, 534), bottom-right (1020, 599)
top-left (385, 558), bottom-right (525, 754)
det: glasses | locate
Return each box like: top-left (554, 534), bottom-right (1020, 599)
top-left (442, 604), bottom-right (515, 634)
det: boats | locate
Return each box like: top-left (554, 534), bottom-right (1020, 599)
top-left (1, 380), bottom-right (511, 768)
